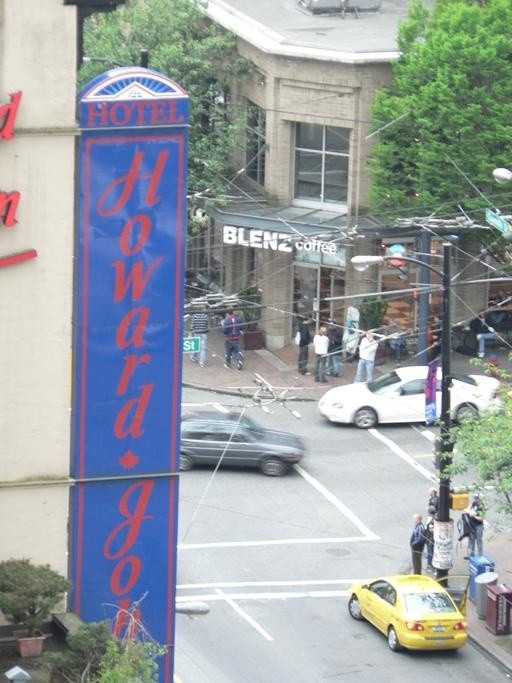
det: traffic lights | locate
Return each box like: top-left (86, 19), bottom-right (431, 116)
top-left (408, 285), bottom-right (419, 299)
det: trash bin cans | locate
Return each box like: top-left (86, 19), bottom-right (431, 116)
top-left (470, 556), bottom-right (512, 636)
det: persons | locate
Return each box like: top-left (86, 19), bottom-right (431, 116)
top-left (294, 318), bottom-right (311, 377)
top-left (425, 507), bottom-right (436, 568)
top-left (410, 514), bottom-right (426, 575)
top-left (220, 309), bottom-right (243, 370)
top-left (387, 323), bottom-right (406, 367)
top-left (189, 309), bottom-right (211, 367)
top-left (325, 321), bottom-right (342, 377)
top-left (463, 494), bottom-right (485, 560)
top-left (428, 488), bottom-right (439, 512)
top-left (477, 305), bottom-right (508, 356)
top-left (353, 334), bottom-right (379, 384)
top-left (430, 315), bottom-right (442, 363)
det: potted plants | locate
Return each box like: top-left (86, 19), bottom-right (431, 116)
top-left (1, 555), bottom-right (73, 658)
top-left (237, 281), bottom-right (265, 350)
top-left (354, 296), bottom-right (388, 365)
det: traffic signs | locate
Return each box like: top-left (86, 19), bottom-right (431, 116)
top-left (183, 338), bottom-right (199, 352)
top-left (485, 210), bottom-right (506, 235)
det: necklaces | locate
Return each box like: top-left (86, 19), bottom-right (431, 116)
top-left (313, 326), bottom-right (330, 383)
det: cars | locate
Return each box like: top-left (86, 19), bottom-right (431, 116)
top-left (179, 411), bottom-right (306, 484)
top-left (450, 306), bottom-right (512, 354)
top-left (319, 366), bottom-right (502, 427)
top-left (344, 576), bottom-right (471, 656)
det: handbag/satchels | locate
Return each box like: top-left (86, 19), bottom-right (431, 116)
top-left (459, 512), bottom-right (475, 540)
top-left (294, 331), bottom-right (301, 345)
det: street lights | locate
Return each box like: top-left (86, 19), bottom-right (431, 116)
top-left (345, 238), bottom-right (458, 589)
top-left (489, 167), bottom-right (512, 245)
